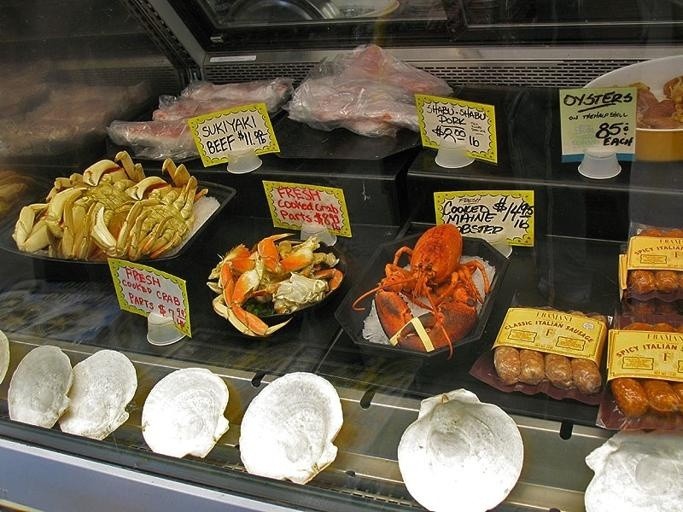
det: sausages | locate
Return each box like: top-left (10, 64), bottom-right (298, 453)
top-left (493, 228), bottom-right (683, 417)
